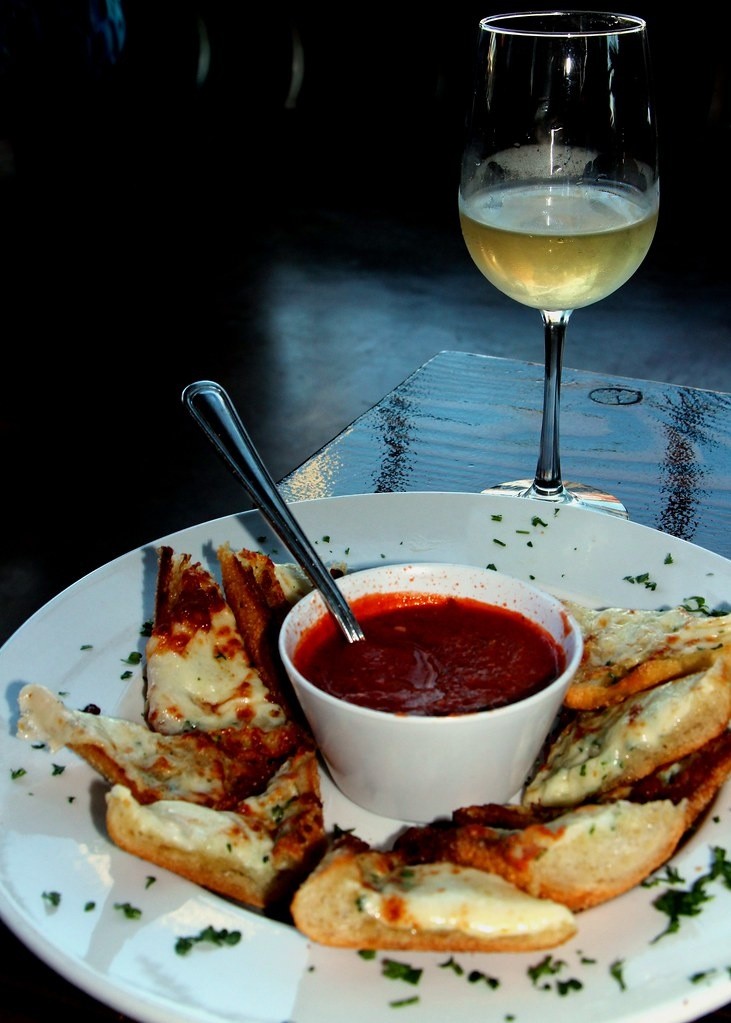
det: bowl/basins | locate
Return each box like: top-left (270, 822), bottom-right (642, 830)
top-left (280, 560), bottom-right (582, 825)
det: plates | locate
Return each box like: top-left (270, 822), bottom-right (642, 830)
top-left (0, 490), bottom-right (730, 1023)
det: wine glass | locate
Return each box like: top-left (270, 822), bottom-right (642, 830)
top-left (455, 12), bottom-right (662, 523)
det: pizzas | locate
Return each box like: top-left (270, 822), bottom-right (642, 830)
top-left (13, 541), bottom-right (731, 954)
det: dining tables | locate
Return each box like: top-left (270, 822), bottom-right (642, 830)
top-left (0, 349), bottom-right (731, 1023)
